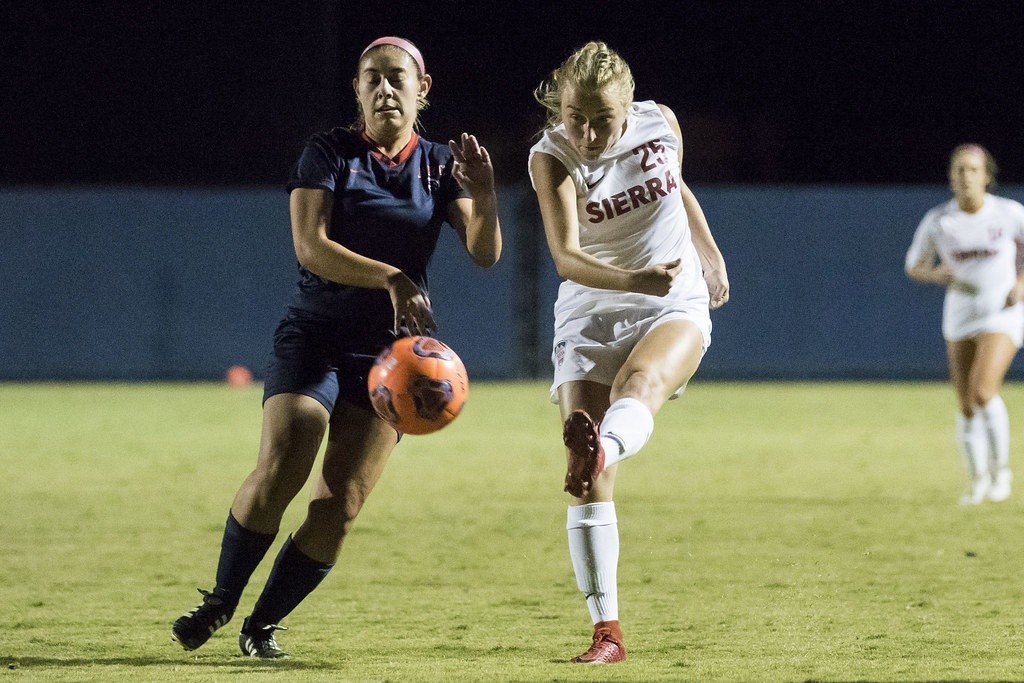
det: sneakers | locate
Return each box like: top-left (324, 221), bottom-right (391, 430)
top-left (174, 588), bottom-right (238, 651)
top-left (570, 627), bottom-right (626, 664)
top-left (563, 409), bottom-right (604, 499)
top-left (240, 617), bottom-right (294, 658)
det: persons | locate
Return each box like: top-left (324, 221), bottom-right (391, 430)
top-left (903, 144), bottom-right (1024, 503)
top-left (172, 36), bottom-right (504, 659)
top-left (528, 41), bottom-right (730, 664)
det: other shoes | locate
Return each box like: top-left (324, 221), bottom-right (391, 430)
top-left (989, 467), bottom-right (1014, 501)
top-left (959, 476), bottom-right (991, 506)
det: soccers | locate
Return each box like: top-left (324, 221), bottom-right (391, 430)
top-left (365, 334), bottom-right (469, 436)
